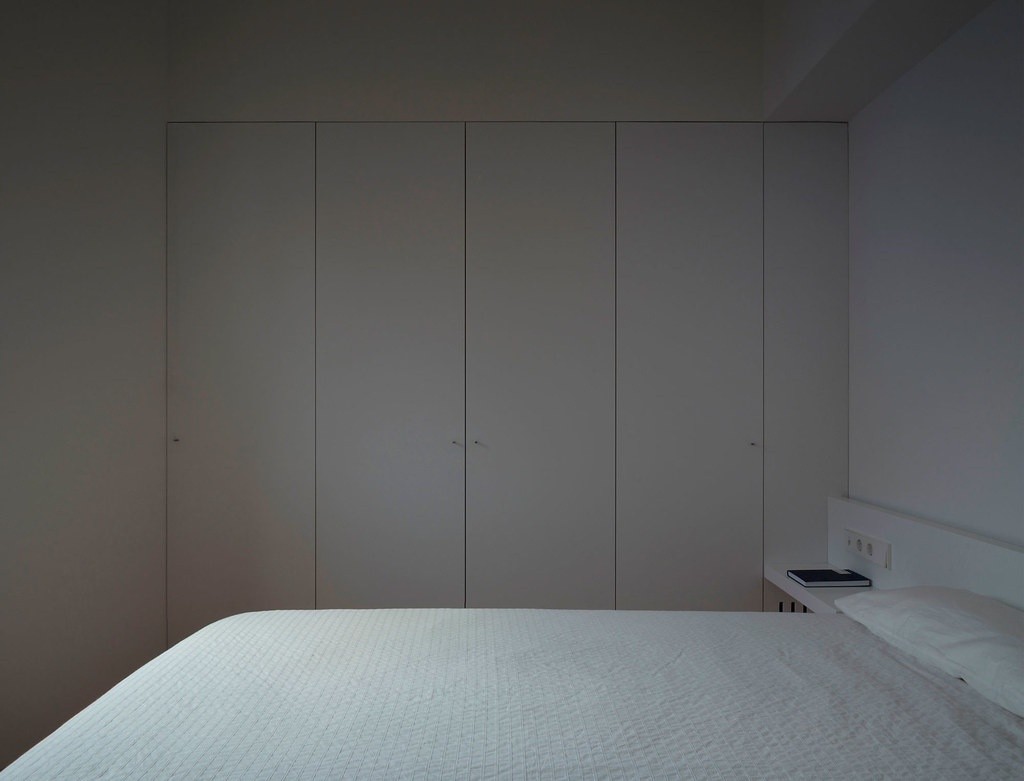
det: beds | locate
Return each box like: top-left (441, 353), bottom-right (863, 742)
top-left (0, 607), bottom-right (1023, 779)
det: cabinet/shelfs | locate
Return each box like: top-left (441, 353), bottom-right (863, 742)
top-left (162, 117), bottom-right (856, 652)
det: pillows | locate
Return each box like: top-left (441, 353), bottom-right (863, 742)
top-left (834, 583), bottom-right (1023, 718)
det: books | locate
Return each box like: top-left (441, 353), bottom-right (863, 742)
top-left (787, 568), bottom-right (872, 587)
